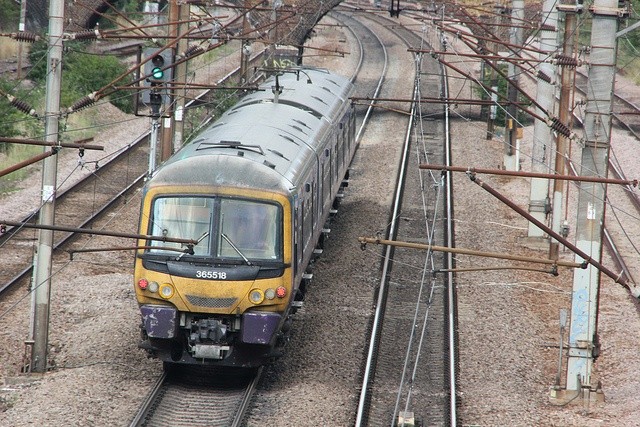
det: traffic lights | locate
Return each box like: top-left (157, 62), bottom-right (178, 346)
top-left (142, 48), bottom-right (172, 107)
